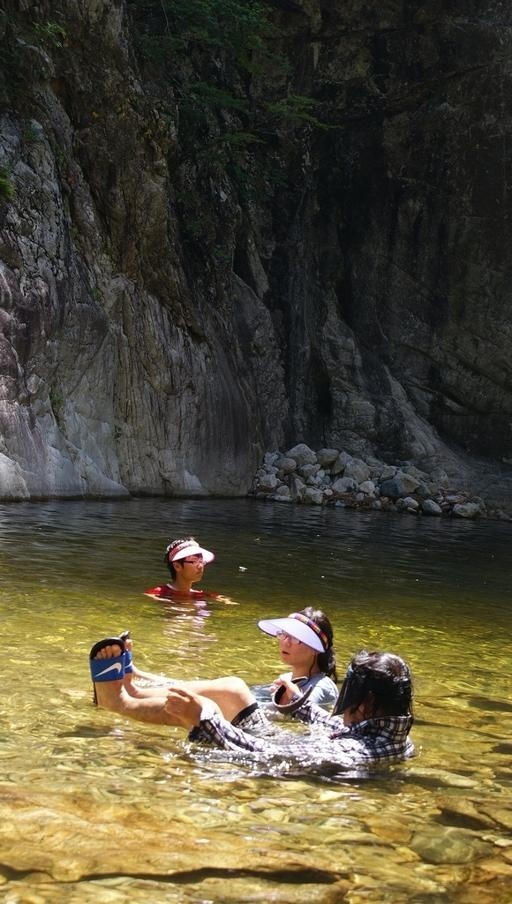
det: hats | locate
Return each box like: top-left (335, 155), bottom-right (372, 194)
top-left (167, 540), bottom-right (215, 563)
top-left (257, 612), bottom-right (328, 654)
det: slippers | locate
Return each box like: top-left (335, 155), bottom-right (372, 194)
top-left (90, 631), bottom-right (134, 705)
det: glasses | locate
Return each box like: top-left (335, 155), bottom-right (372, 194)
top-left (183, 561), bottom-right (207, 566)
top-left (276, 631), bottom-right (301, 645)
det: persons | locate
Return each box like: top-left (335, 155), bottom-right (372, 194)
top-left (144, 536), bottom-right (232, 608)
top-left (90, 630), bottom-right (413, 772)
top-left (255, 607), bottom-right (339, 706)
